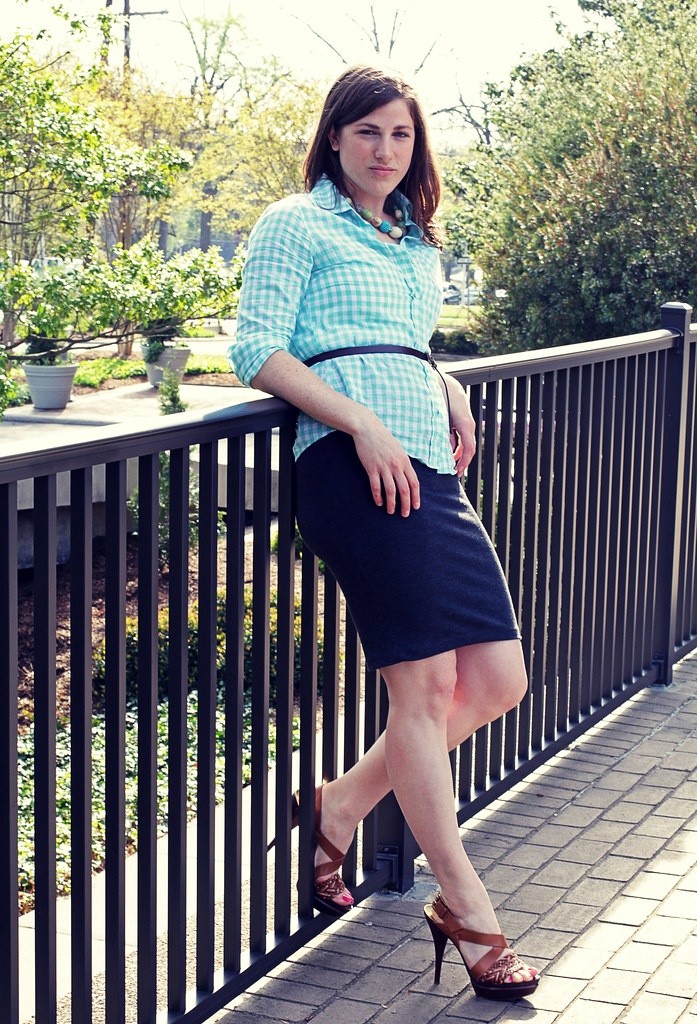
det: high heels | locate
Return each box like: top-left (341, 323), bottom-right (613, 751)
top-left (423, 892), bottom-right (541, 1000)
top-left (267, 784), bottom-right (351, 917)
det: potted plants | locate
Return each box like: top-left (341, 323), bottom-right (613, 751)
top-left (0, 242), bottom-right (246, 412)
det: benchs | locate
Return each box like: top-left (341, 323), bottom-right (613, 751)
top-left (164, 432), bottom-right (279, 512)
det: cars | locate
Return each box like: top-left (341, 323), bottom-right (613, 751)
top-left (442, 284), bottom-right (462, 307)
top-left (464, 290), bottom-right (483, 306)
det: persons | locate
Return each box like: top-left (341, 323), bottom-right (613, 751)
top-left (227, 65), bottom-right (542, 999)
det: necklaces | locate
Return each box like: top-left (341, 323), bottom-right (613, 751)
top-left (346, 197), bottom-right (404, 238)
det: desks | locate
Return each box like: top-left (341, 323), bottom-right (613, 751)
top-left (0, 381), bottom-right (272, 570)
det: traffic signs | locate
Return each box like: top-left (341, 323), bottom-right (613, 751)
top-left (456, 257), bottom-right (473, 263)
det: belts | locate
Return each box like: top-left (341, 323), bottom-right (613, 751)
top-left (303, 345), bottom-right (452, 435)
